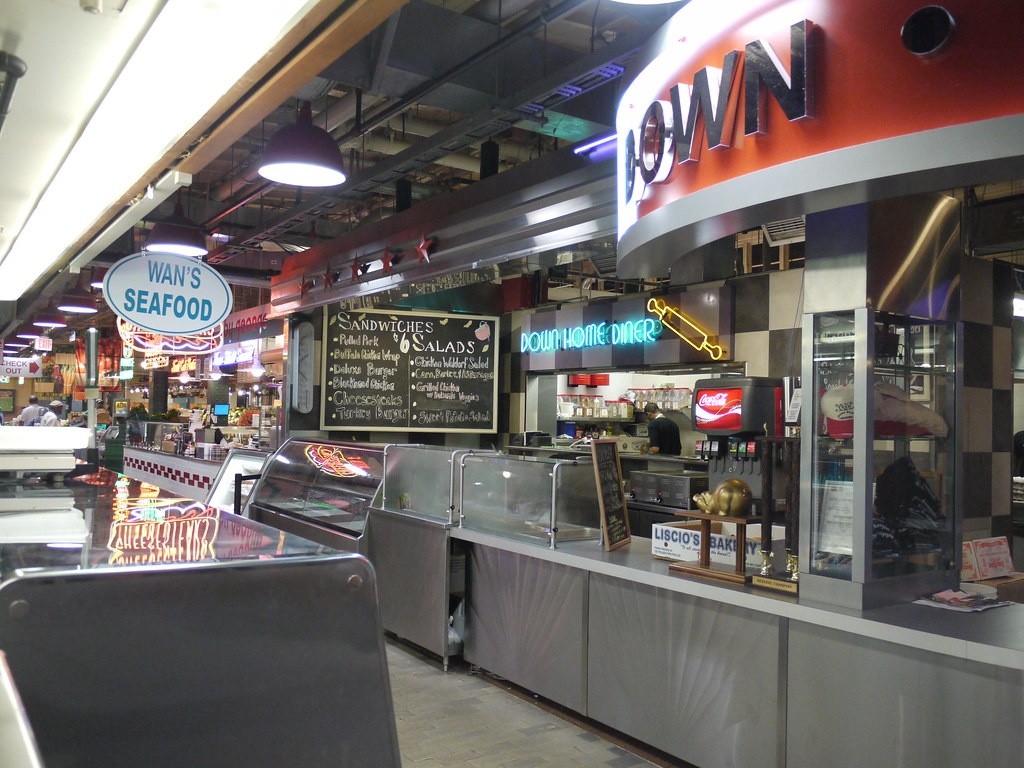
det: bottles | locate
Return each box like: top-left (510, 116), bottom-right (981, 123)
top-left (576, 424), bottom-right (613, 440)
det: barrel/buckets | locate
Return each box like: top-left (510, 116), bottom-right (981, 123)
top-left (557, 394), bottom-right (634, 419)
top-left (565, 421), bottom-right (576, 437)
top-left (626, 387), bottom-right (693, 411)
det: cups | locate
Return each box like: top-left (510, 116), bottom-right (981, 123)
top-left (561, 402), bottom-right (573, 418)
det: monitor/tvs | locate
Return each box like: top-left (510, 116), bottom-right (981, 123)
top-left (99, 426), bottom-right (119, 445)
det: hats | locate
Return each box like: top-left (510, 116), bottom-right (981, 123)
top-left (49, 399), bottom-right (63, 407)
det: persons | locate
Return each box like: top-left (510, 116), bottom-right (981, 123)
top-left (644, 403), bottom-right (682, 456)
top-left (19, 396), bottom-right (64, 426)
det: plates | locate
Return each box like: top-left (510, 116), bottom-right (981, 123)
top-left (211, 444), bottom-right (227, 461)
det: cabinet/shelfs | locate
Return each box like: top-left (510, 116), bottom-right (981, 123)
top-left (798, 307), bottom-right (966, 610)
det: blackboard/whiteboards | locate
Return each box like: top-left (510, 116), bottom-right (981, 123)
top-left (321, 305), bottom-right (498, 431)
top-left (591, 438), bottom-right (632, 553)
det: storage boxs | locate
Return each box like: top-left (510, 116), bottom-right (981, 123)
top-left (650, 520), bottom-right (787, 574)
top-left (629, 423), bottom-right (650, 437)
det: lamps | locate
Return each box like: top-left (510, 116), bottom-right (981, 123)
top-left (130, 282), bottom-right (266, 395)
top-left (256, 102), bottom-right (346, 188)
top-left (2, 267), bottom-right (109, 356)
top-left (146, 189), bottom-right (209, 256)
